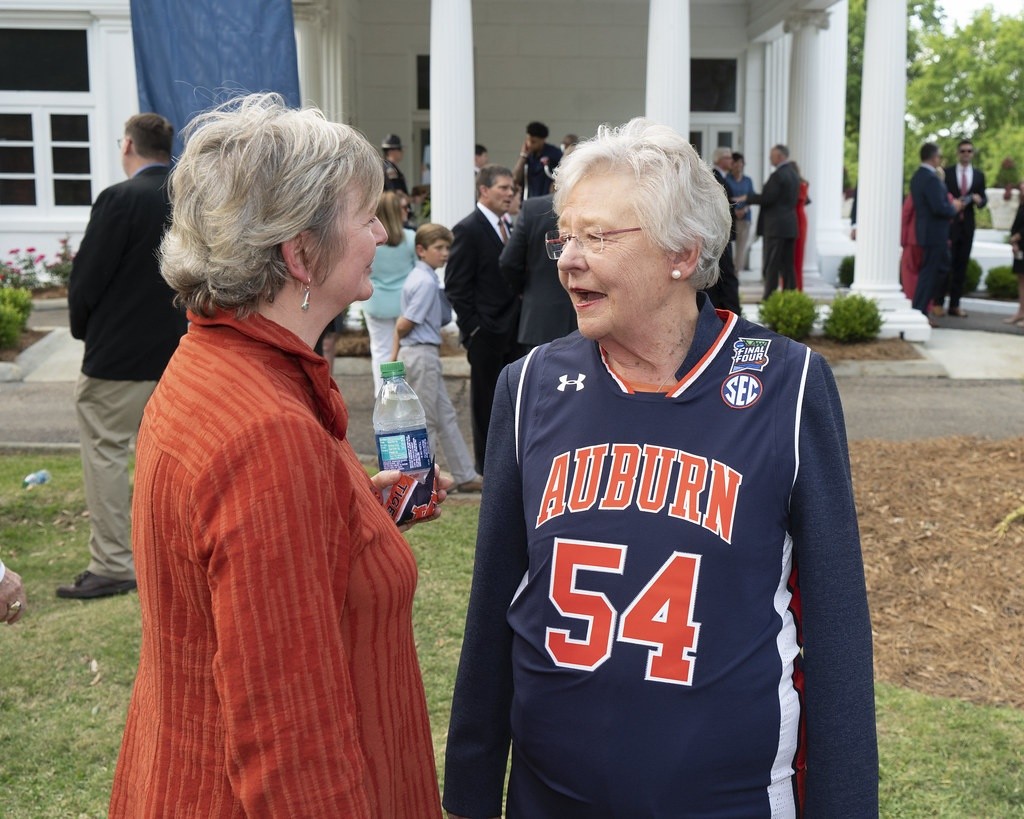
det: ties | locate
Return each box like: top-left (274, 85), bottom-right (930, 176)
top-left (962, 165), bottom-right (967, 197)
top-left (498, 219), bottom-right (508, 243)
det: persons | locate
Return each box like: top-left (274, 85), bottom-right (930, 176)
top-left (111, 92), bottom-right (453, 819)
top-left (390, 224), bottom-right (483, 494)
top-left (704, 145), bottom-right (809, 317)
top-left (0, 558), bottom-right (27, 625)
top-left (56, 113), bottom-right (187, 598)
top-left (445, 122), bottom-right (578, 476)
top-left (441, 118), bottom-right (879, 819)
top-left (314, 133), bottom-right (431, 399)
top-left (901, 140), bottom-right (1024, 327)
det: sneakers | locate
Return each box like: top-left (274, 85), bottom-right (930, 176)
top-left (56, 570), bottom-right (137, 599)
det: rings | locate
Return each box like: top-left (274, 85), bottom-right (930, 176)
top-left (9, 601), bottom-right (21, 611)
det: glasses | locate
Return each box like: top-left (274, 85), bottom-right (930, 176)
top-left (544, 225), bottom-right (642, 260)
top-left (960, 149), bottom-right (973, 153)
top-left (117, 138), bottom-right (126, 149)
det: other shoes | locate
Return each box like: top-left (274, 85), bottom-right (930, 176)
top-left (1003, 316), bottom-right (1024, 327)
top-left (457, 477), bottom-right (485, 492)
top-left (949, 309), bottom-right (967, 318)
top-left (445, 482), bottom-right (457, 494)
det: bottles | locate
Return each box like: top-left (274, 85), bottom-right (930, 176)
top-left (23, 469), bottom-right (49, 487)
top-left (372, 360), bottom-right (436, 524)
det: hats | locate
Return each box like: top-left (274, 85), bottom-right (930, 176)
top-left (381, 133), bottom-right (403, 149)
